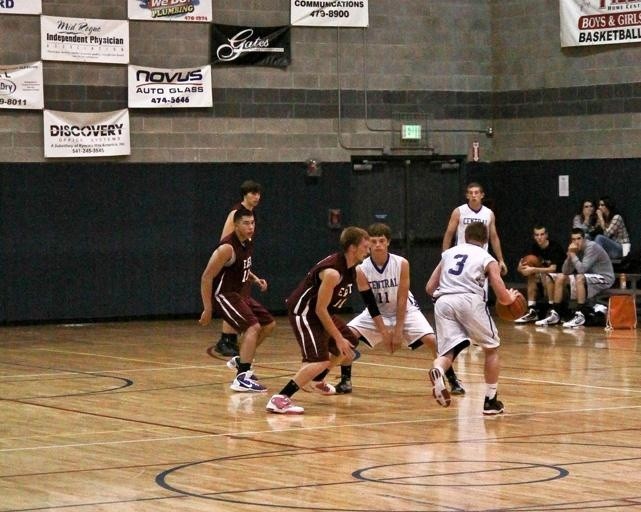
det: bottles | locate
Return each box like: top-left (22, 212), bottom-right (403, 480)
top-left (620, 273), bottom-right (628, 289)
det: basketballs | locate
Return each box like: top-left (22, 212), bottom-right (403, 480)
top-left (522, 254), bottom-right (540, 268)
top-left (494, 290), bottom-right (528, 323)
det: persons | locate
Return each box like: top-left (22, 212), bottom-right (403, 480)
top-left (514, 223), bottom-right (566, 327)
top-left (198, 208), bottom-right (275, 393)
top-left (535, 228), bottom-right (615, 328)
top-left (595, 199), bottom-right (632, 259)
top-left (424, 222), bottom-right (522, 416)
top-left (334, 222), bottom-right (467, 395)
top-left (443, 182), bottom-right (508, 277)
top-left (213, 178), bottom-right (265, 357)
top-left (573, 200), bottom-right (596, 241)
top-left (267, 225), bottom-right (372, 415)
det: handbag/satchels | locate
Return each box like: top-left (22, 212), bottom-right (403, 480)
top-left (606, 296), bottom-right (638, 331)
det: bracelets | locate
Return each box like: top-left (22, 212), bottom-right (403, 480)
top-left (600, 218), bottom-right (604, 220)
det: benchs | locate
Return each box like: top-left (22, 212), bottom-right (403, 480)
top-left (547, 268), bottom-right (641, 328)
top-left (502, 280), bottom-right (640, 327)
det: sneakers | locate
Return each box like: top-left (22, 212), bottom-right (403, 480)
top-left (215, 338), bottom-right (267, 391)
top-left (483, 392), bottom-right (504, 413)
top-left (563, 310), bottom-right (586, 326)
top-left (514, 308), bottom-right (539, 322)
top-left (535, 310), bottom-right (560, 325)
top-left (266, 395), bottom-right (304, 413)
top-left (428, 368), bottom-right (465, 407)
top-left (312, 380), bottom-right (352, 394)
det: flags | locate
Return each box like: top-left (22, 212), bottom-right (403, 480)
top-left (559, 0), bottom-right (641, 48)
top-left (128, 1), bottom-right (212, 22)
top-left (0, 0), bottom-right (43, 15)
top-left (127, 65), bottom-right (213, 111)
top-left (292, 0), bottom-right (368, 28)
top-left (41, 15), bottom-right (129, 64)
top-left (44, 110), bottom-right (130, 157)
top-left (211, 23), bottom-right (291, 66)
top-left (0, 61), bottom-right (44, 109)
